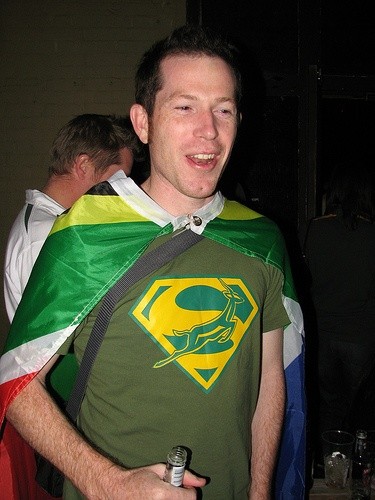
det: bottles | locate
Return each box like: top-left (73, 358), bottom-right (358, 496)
top-left (306, 419), bottom-right (315, 491)
top-left (351, 430), bottom-right (372, 500)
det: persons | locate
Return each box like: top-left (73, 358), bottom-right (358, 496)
top-left (0, 23), bottom-right (299, 500)
top-left (2, 113), bottom-right (137, 328)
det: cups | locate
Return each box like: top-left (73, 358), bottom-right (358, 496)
top-left (321, 430), bottom-right (355, 491)
